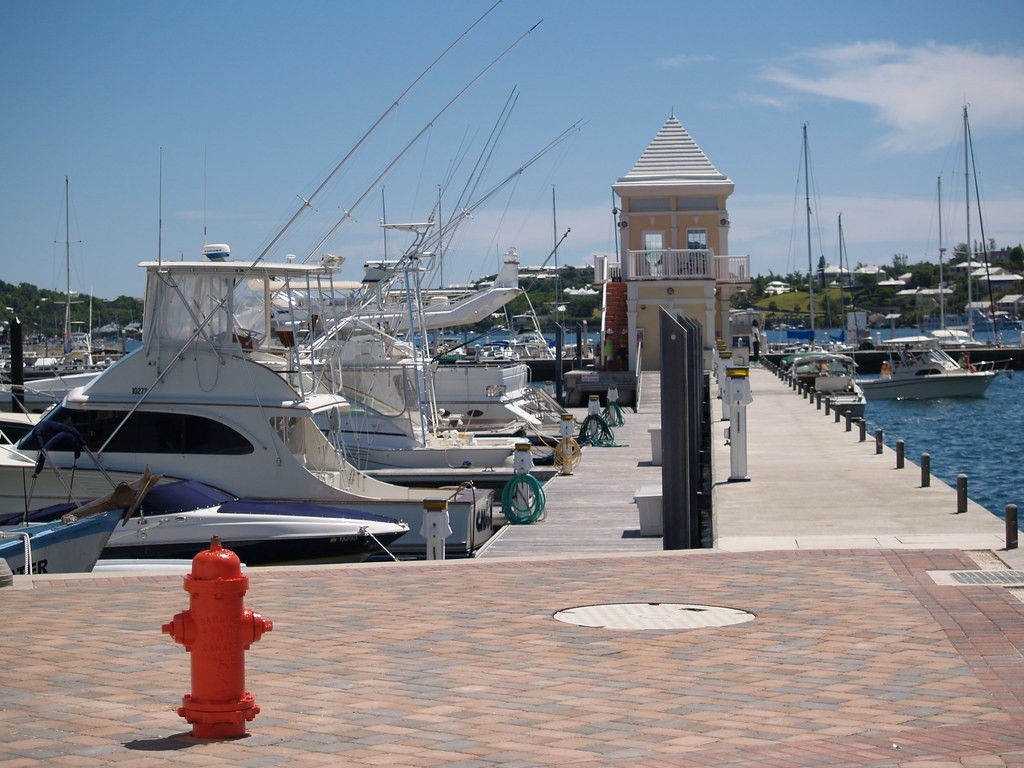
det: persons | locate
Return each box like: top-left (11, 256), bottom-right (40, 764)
top-left (750, 319), bottom-right (761, 366)
top-left (901, 344), bottom-right (912, 367)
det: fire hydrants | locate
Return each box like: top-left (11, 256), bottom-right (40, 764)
top-left (159, 534), bottom-right (274, 741)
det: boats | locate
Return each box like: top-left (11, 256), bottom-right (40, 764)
top-left (0, 0), bottom-right (592, 573)
top-left (852, 337), bottom-right (1015, 402)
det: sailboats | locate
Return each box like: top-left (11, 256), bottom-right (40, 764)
top-left (766, 104), bottom-right (1023, 421)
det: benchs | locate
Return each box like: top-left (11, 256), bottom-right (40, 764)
top-left (648, 423), bottom-right (662, 465)
top-left (633, 485), bottom-right (664, 535)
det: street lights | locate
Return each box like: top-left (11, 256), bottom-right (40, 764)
top-left (35, 305), bottom-right (48, 359)
top-left (41, 298), bottom-right (57, 338)
